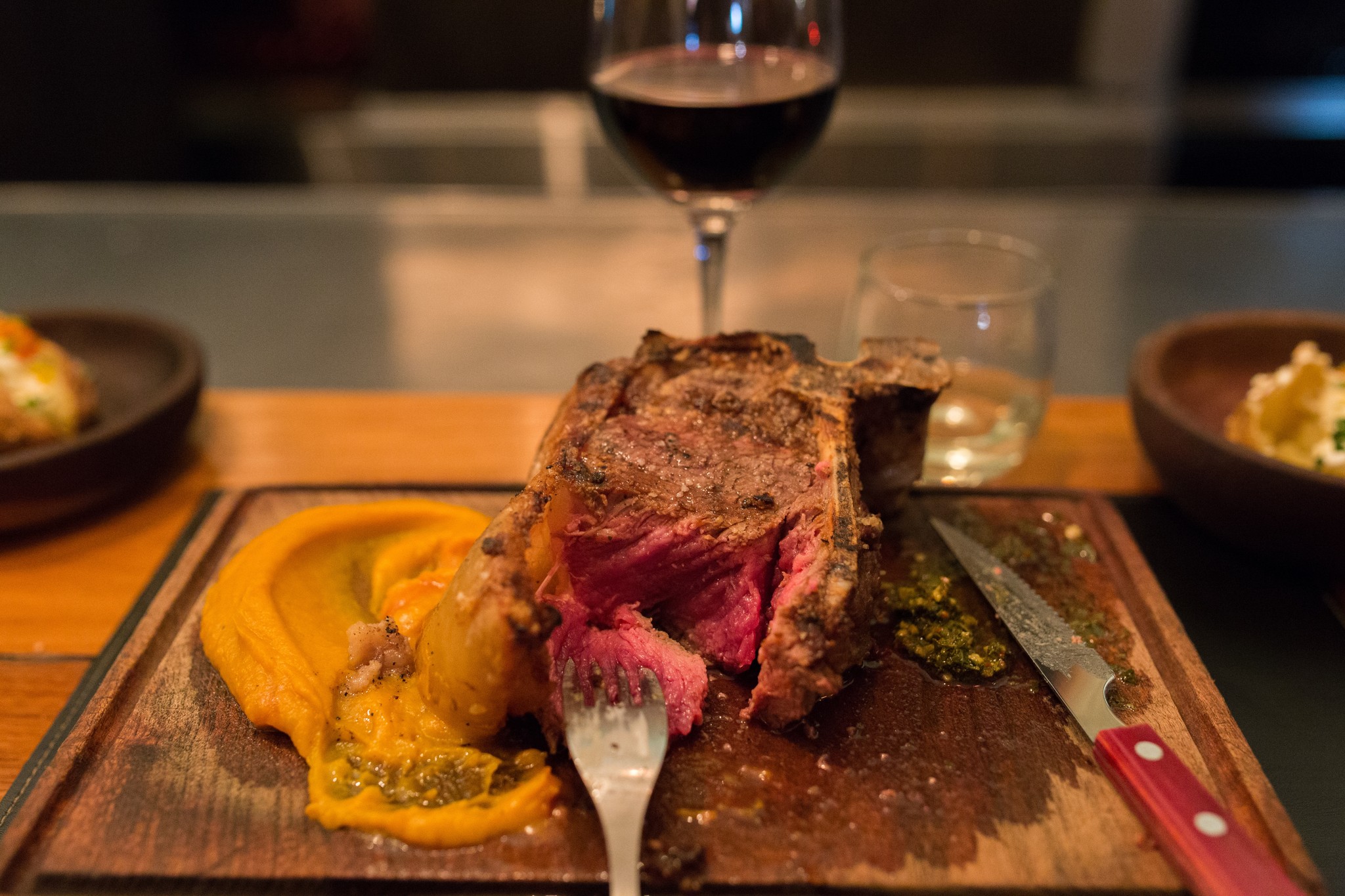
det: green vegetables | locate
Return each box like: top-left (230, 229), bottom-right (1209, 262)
top-left (873, 509), bottom-right (1098, 685)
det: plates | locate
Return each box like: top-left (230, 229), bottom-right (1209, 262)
top-left (0, 485), bottom-right (1329, 896)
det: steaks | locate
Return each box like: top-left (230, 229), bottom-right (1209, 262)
top-left (525, 326), bottom-right (950, 736)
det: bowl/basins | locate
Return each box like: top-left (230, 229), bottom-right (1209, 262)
top-left (0, 307), bottom-right (203, 539)
top-left (1127, 307), bottom-right (1345, 548)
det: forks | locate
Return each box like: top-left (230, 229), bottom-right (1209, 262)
top-left (560, 656), bottom-right (670, 896)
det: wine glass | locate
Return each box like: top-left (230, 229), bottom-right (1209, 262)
top-left (585, 0), bottom-right (845, 338)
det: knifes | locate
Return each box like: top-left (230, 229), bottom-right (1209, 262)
top-left (927, 513), bottom-right (1310, 895)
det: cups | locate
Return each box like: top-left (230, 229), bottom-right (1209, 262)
top-left (836, 229), bottom-right (1057, 489)
